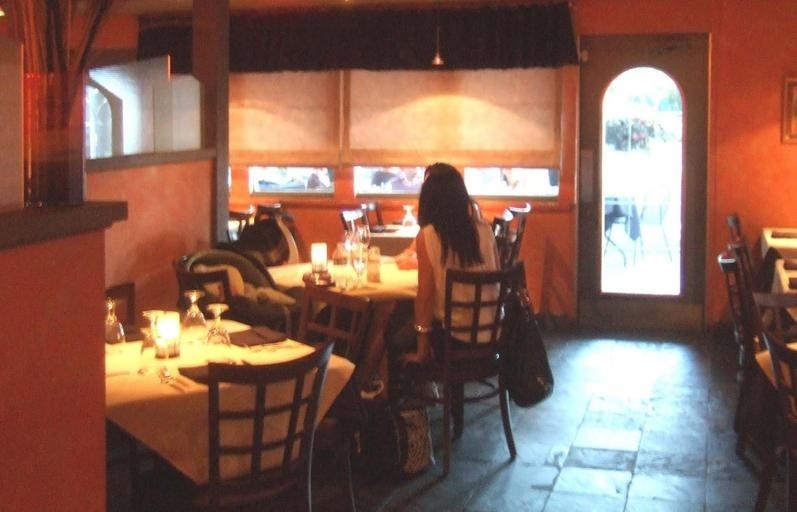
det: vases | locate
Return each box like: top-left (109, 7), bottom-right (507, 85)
top-left (22, 71), bottom-right (87, 207)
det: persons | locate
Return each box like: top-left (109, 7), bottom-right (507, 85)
top-left (387, 172), bottom-right (501, 476)
top-left (397, 162), bottom-right (482, 271)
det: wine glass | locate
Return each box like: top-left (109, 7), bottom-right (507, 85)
top-left (105, 290), bottom-right (231, 359)
top-left (333, 222), bottom-right (382, 289)
top-left (400, 204), bottom-right (419, 234)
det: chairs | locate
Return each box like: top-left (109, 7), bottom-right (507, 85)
top-left (716, 212), bottom-right (797, 510)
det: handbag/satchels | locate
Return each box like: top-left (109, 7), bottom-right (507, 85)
top-left (388, 404), bottom-right (437, 480)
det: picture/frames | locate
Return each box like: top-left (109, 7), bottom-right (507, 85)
top-left (781, 73), bottom-right (797, 145)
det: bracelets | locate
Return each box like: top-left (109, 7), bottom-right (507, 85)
top-left (412, 323), bottom-right (431, 338)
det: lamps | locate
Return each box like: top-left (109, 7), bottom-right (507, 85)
top-left (431, 1), bottom-right (444, 66)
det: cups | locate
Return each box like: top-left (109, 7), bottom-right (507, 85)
top-left (310, 242), bottom-right (329, 274)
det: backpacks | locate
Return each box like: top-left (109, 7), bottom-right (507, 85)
top-left (498, 287), bottom-right (555, 408)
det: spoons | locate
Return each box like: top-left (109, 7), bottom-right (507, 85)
top-left (154, 367), bottom-right (190, 395)
